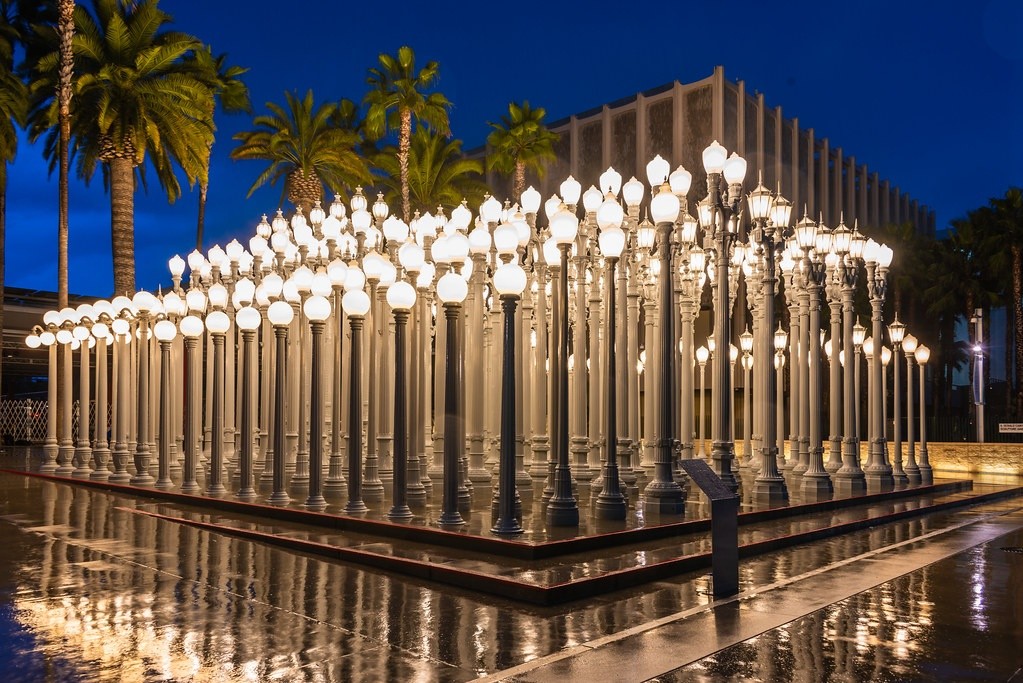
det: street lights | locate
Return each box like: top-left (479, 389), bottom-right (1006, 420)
top-left (24, 138), bottom-right (934, 537)
top-left (971, 308), bottom-right (985, 443)
